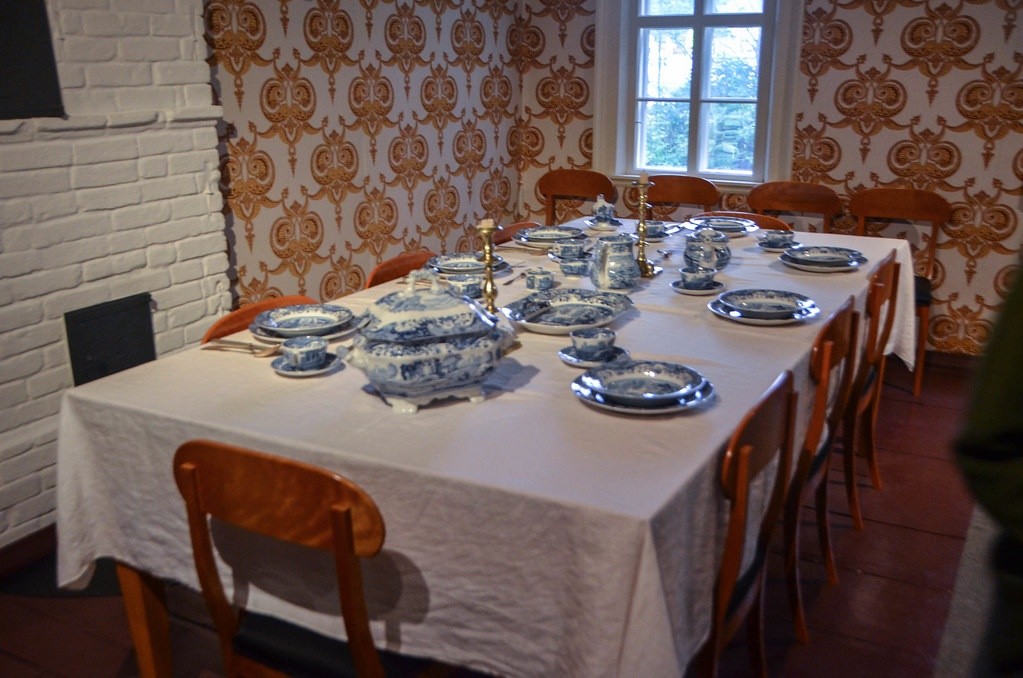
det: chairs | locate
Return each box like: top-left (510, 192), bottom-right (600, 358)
top-left (203, 170), bottom-right (954, 400)
top-left (703, 249), bottom-right (901, 678)
top-left (169, 441), bottom-right (388, 678)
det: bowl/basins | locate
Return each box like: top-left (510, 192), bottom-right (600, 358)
top-left (560, 257), bottom-right (594, 277)
top-left (553, 238), bottom-right (589, 259)
top-left (524, 267), bottom-right (555, 293)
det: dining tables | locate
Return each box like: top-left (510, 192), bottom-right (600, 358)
top-left (56, 216), bottom-right (915, 678)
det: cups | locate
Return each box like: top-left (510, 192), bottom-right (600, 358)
top-left (570, 327), bottom-right (617, 363)
top-left (679, 265), bottom-right (717, 290)
top-left (764, 230), bottom-right (795, 248)
top-left (282, 335), bottom-right (329, 371)
top-left (636, 220), bottom-right (664, 237)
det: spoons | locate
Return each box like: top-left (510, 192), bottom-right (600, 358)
top-left (201, 346), bottom-right (279, 357)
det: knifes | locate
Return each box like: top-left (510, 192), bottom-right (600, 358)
top-left (503, 269), bottom-right (532, 285)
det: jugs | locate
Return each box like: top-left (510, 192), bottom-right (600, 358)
top-left (683, 227), bottom-right (733, 270)
top-left (592, 193), bottom-right (614, 220)
top-left (589, 231), bottom-right (641, 290)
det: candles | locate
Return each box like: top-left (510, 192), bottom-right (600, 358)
top-left (639, 173), bottom-right (649, 183)
top-left (480, 219), bottom-right (494, 226)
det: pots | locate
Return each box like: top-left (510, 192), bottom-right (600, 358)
top-left (334, 268), bottom-right (516, 415)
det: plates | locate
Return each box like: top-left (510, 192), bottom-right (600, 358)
top-left (269, 351), bottom-right (342, 377)
top-left (558, 345), bottom-right (629, 369)
top-left (668, 279), bottom-right (726, 295)
top-left (516, 286), bottom-right (634, 334)
top-left (423, 253), bottom-right (510, 281)
top-left (632, 232), bottom-right (672, 242)
top-left (680, 216), bottom-right (760, 238)
top-left (511, 226), bottom-right (588, 249)
top-left (756, 241), bottom-right (804, 253)
top-left (706, 289), bottom-right (820, 326)
top-left (777, 247), bottom-right (868, 273)
top-left (570, 361), bottom-right (716, 417)
top-left (247, 304), bottom-right (371, 343)
